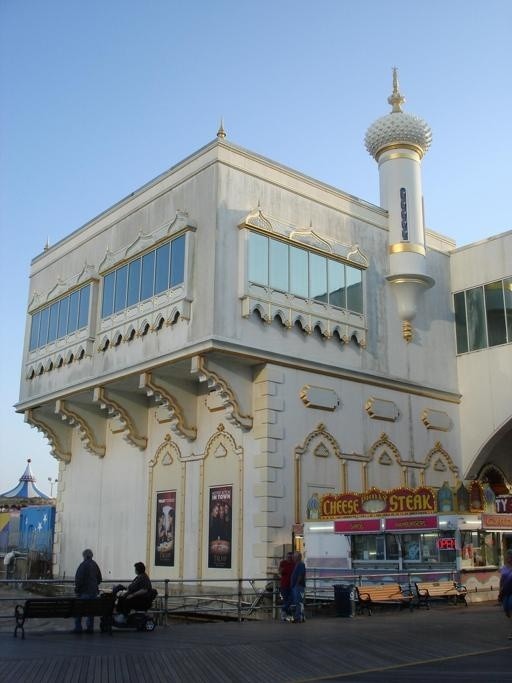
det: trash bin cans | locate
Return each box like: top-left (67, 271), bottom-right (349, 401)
top-left (332, 584), bottom-right (356, 618)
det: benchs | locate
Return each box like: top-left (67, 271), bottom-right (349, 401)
top-left (352, 580), bottom-right (468, 615)
top-left (11, 595), bottom-right (116, 640)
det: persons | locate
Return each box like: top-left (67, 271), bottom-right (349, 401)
top-left (290, 552), bottom-right (306, 623)
top-left (112, 562), bottom-right (152, 624)
top-left (72, 549), bottom-right (102, 632)
top-left (279, 553), bottom-right (295, 622)
top-left (498, 549), bottom-right (512, 640)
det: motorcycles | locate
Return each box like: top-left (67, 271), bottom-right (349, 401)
top-left (99, 582), bottom-right (159, 632)
